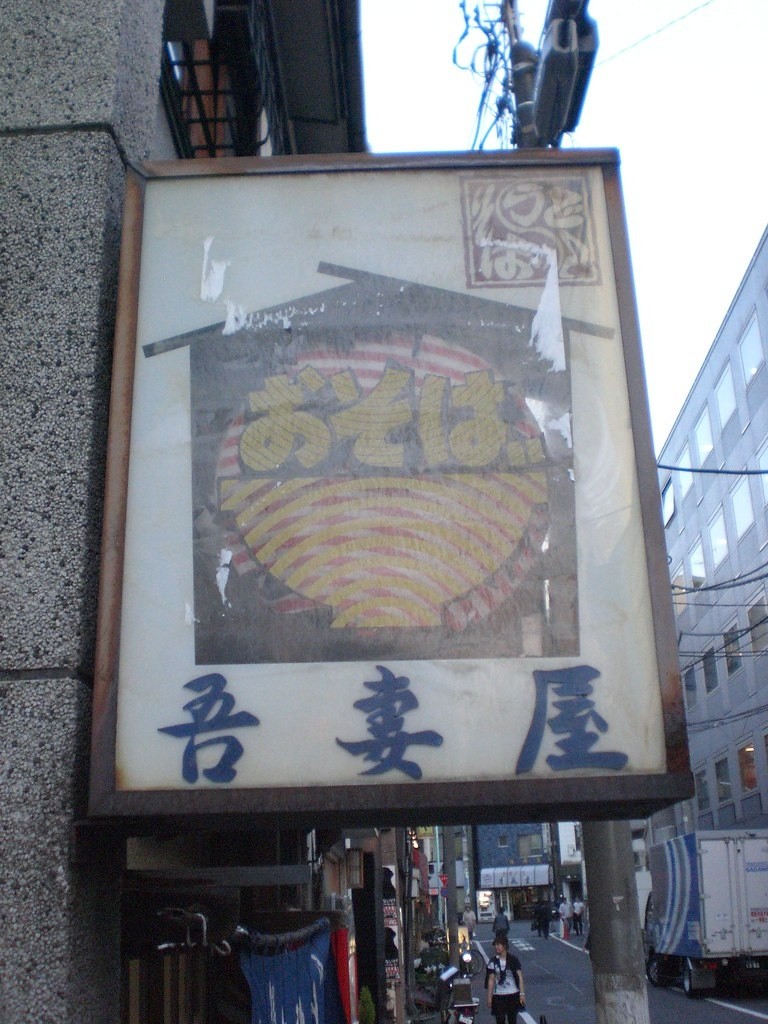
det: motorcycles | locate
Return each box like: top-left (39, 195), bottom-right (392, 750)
top-left (451, 1002), bottom-right (480, 1024)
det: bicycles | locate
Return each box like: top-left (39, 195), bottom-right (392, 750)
top-left (458, 943), bottom-right (485, 974)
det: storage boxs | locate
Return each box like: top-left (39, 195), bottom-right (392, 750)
top-left (453, 979), bottom-right (473, 1004)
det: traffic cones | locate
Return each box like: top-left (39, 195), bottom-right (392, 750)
top-left (563, 926), bottom-right (569, 940)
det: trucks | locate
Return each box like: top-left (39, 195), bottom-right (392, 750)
top-left (636, 829), bottom-right (768, 999)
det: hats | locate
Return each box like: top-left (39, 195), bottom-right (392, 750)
top-left (492, 935), bottom-right (509, 948)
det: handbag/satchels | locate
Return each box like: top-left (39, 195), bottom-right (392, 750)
top-left (531, 921), bottom-right (537, 931)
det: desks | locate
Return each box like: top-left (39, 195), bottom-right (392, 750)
top-left (455, 997), bottom-right (480, 1024)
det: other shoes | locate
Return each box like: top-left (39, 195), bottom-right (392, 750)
top-left (470, 939), bottom-right (474, 945)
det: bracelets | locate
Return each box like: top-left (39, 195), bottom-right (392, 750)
top-left (519, 993), bottom-right (525, 995)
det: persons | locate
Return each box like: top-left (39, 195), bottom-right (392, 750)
top-left (558, 897), bottom-right (574, 932)
top-left (492, 908), bottom-right (510, 938)
top-left (573, 895), bottom-right (584, 934)
top-left (532, 900), bottom-right (551, 938)
top-left (484, 936), bottom-right (526, 1024)
top-left (462, 905), bottom-right (476, 939)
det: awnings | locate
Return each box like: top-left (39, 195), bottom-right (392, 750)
top-left (480, 865), bottom-right (553, 888)
